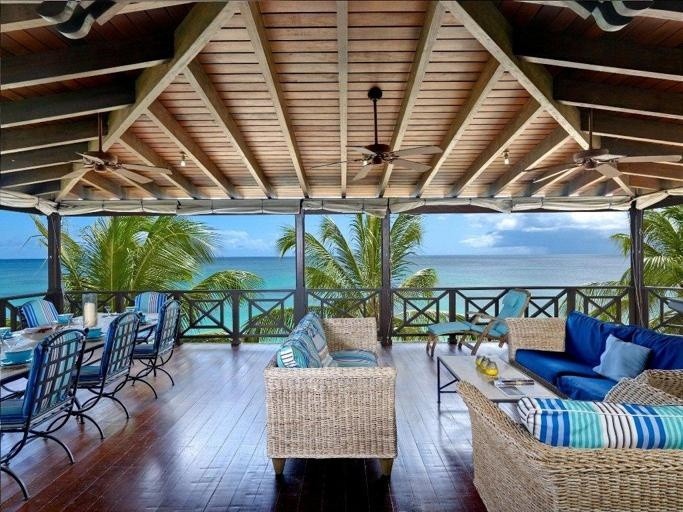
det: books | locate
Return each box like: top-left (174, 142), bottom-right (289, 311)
top-left (488, 377), bottom-right (550, 397)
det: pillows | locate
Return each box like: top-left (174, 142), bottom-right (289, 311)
top-left (592, 334), bottom-right (652, 383)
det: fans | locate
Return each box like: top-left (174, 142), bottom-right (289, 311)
top-left (519, 96), bottom-right (683, 196)
top-left (309, 85), bottom-right (443, 188)
top-left (61, 114), bottom-right (171, 186)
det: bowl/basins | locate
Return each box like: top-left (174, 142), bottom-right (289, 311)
top-left (0, 326), bottom-right (12, 337)
top-left (86, 327), bottom-right (102, 340)
top-left (5, 349), bottom-right (32, 362)
top-left (58, 313), bottom-right (74, 323)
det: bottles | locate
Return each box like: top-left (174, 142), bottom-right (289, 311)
top-left (475, 354), bottom-right (498, 376)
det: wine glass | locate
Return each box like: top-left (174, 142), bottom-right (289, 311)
top-left (104, 306), bottom-right (112, 319)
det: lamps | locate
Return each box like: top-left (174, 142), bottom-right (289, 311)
top-left (502, 148), bottom-right (509, 165)
top-left (181, 152), bottom-right (185, 168)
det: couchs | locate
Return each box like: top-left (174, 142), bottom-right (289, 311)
top-left (263, 311), bottom-right (398, 476)
top-left (503, 309), bottom-right (683, 402)
top-left (455, 378), bottom-right (683, 512)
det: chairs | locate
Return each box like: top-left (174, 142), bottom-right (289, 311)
top-left (426, 286), bottom-right (531, 359)
top-left (0, 327), bottom-right (90, 501)
top-left (132, 299), bottom-right (182, 386)
top-left (16, 299), bottom-right (58, 329)
top-left (68, 311), bottom-right (140, 440)
top-left (133, 291), bottom-right (167, 313)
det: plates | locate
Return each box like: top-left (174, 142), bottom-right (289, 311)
top-left (0, 358), bottom-right (31, 367)
